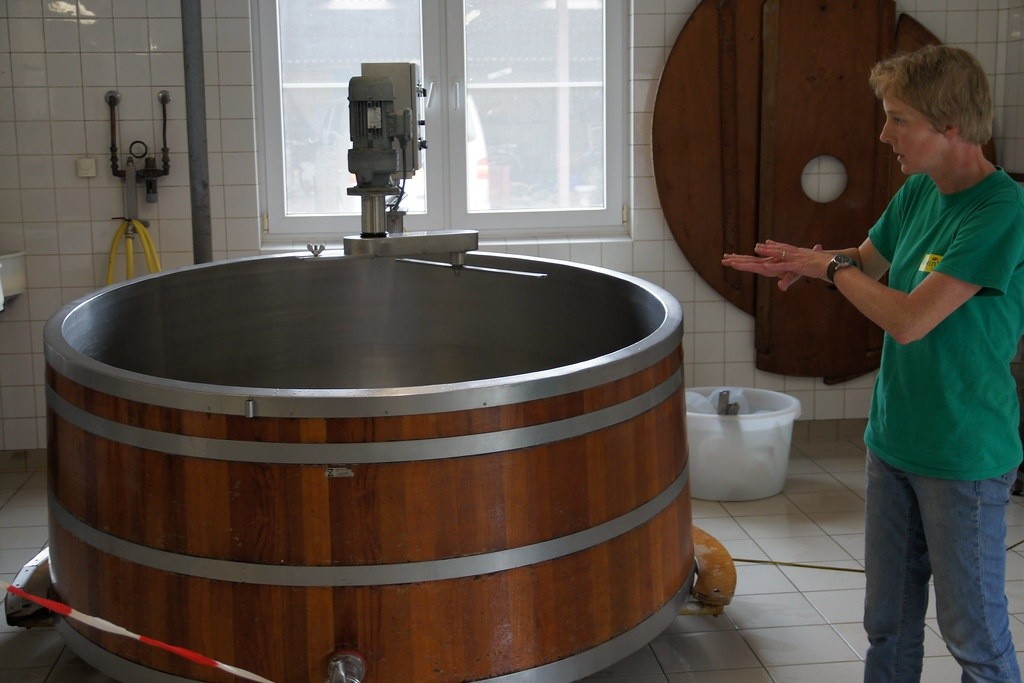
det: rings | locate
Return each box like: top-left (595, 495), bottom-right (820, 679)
top-left (781, 250), bottom-right (786, 260)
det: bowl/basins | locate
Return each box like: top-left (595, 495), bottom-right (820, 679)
top-left (708, 388), bottom-right (749, 415)
top-left (686, 392), bottom-right (714, 414)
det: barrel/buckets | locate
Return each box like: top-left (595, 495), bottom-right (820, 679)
top-left (42, 239), bottom-right (696, 683)
top-left (685, 387), bottom-right (801, 502)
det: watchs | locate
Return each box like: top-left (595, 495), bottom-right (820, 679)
top-left (827, 255), bottom-right (857, 291)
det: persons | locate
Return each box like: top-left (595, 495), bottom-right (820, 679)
top-left (721, 46), bottom-right (1024, 683)
